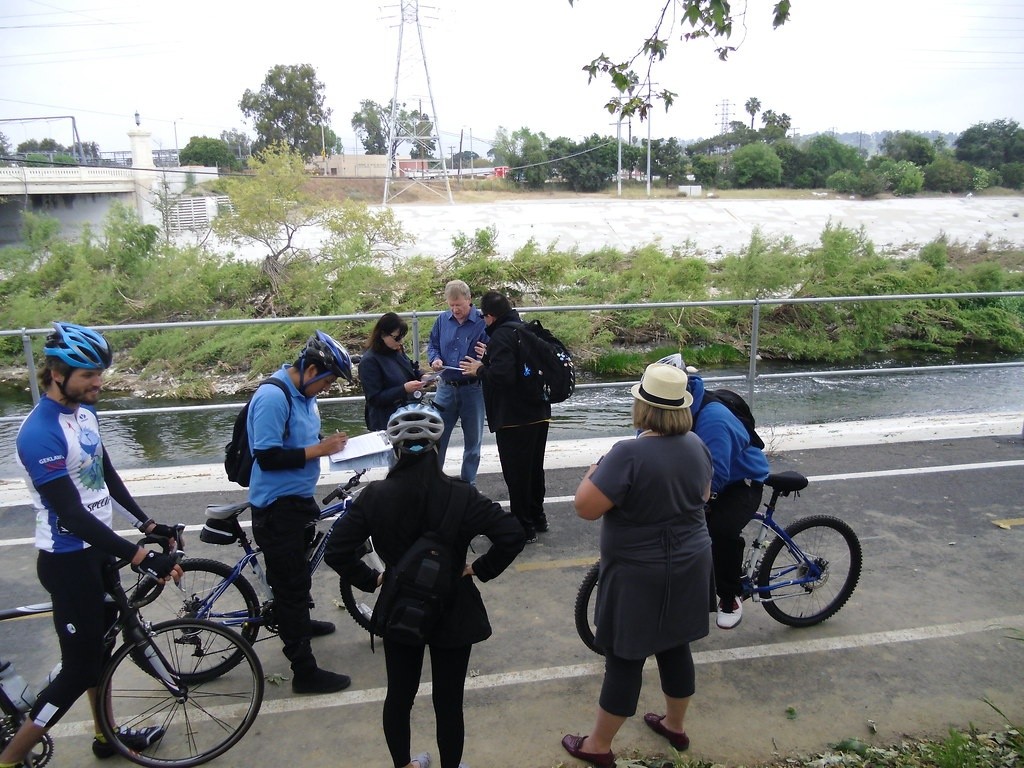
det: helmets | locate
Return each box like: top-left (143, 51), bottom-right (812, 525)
top-left (387, 404), bottom-right (444, 455)
top-left (655, 353), bottom-right (688, 374)
top-left (44, 322), bottom-right (113, 370)
top-left (304, 330), bottom-right (352, 382)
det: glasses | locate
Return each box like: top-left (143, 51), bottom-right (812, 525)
top-left (479, 313), bottom-right (487, 319)
top-left (385, 330), bottom-right (402, 342)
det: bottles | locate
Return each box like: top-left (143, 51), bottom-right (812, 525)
top-left (33, 662), bottom-right (63, 701)
top-left (0, 659), bottom-right (33, 712)
top-left (361, 604), bottom-right (374, 619)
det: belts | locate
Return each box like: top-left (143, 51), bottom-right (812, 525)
top-left (445, 378), bottom-right (476, 387)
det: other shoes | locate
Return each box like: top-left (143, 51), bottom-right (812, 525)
top-left (411, 752), bottom-right (430, 768)
top-left (525, 528), bottom-right (540, 543)
top-left (535, 513), bottom-right (550, 532)
top-left (458, 762), bottom-right (465, 768)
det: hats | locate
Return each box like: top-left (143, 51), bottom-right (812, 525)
top-left (631, 363), bottom-right (694, 410)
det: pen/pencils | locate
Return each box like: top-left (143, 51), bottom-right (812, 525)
top-left (335, 428), bottom-right (339, 433)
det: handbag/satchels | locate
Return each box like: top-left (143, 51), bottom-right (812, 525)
top-left (373, 531), bottom-right (449, 648)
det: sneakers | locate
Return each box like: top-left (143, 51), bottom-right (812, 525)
top-left (93, 726), bottom-right (165, 758)
top-left (717, 595), bottom-right (742, 630)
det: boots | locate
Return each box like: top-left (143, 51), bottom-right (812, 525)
top-left (304, 604), bottom-right (335, 635)
top-left (283, 637), bottom-right (351, 693)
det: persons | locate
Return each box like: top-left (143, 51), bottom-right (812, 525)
top-left (0, 319), bottom-right (183, 768)
top-left (460, 289), bottom-right (553, 543)
top-left (322, 404), bottom-right (527, 768)
top-left (428, 279), bottom-right (489, 484)
top-left (645, 353), bottom-right (770, 629)
top-left (561, 364), bottom-right (716, 768)
top-left (248, 329), bottom-right (353, 694)
top-left (359, 312), bottom-right (436, 460)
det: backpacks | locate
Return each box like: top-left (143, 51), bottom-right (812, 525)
top-left (693, 389), bottom-right (765, 450)
top-left (224, 377), bottom-right (292, 487)
top-left (500, 319), bottom-right (575, 403)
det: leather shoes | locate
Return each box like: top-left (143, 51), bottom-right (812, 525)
top-left (562, 734), bottom-right (614, 768)
top-left (643, 713), bottom-right (690, 750)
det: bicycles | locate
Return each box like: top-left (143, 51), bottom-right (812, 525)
top-left (572, 468), bottom-right (866, 658)
top-left (122, 465), bottom-right (388, 683)
top-left (1, 521), bottom-right (266, 768)
